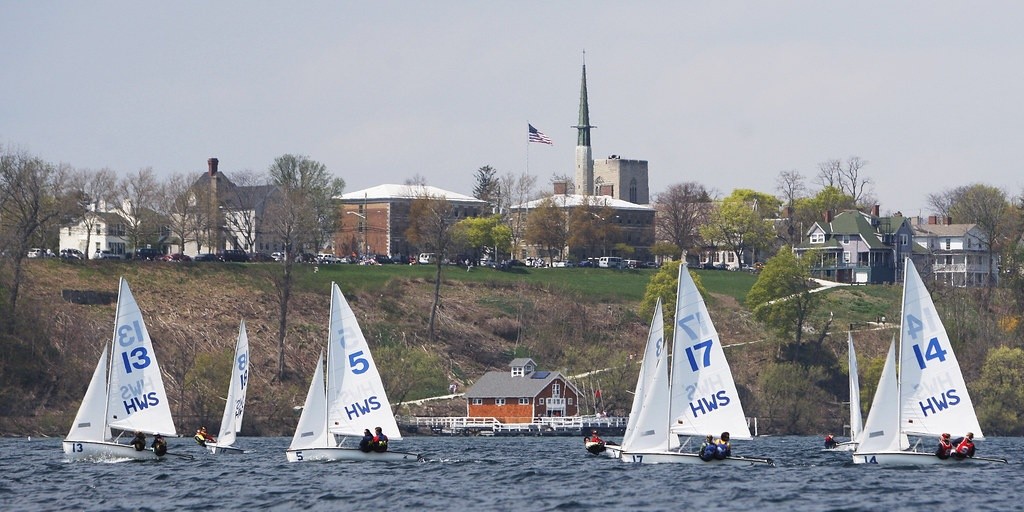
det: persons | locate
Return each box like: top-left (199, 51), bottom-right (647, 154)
top-left (540, 259), bottom-right (545, 266)
top-left (699, 432), bottom-right (731, 456)
top-left (950, 433), bottom-right (976, 460)
top-left (162, 257), bottom-right (172, 262)
top-left (46, 246), bottom-right (55, 258)
top-left (584, 429), bottom-right (620, 455)
top-left (150, 431), bottom-right (164, 455)
top-left (358, 427), bottom-right (388, 452)
top-left (824, 433), bottom-right (839, 449)
top-left (465, 259), bottom-right (469, 265)
top-left (369, 258), bottom-right (376, 264)
top-left (194, 426), bottom-right (216, 447)
top-left (130, 431), bottom-right (146, 451)
top-left (935, 433), bottom-right (953, 460)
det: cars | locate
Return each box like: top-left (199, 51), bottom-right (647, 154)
top-left (26, 247), bottom-right (753, 270)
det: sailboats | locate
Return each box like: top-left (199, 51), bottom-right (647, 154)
top-left (204, 319), bottom-right (250, 455)
top-left (605, 263), bottom-right (775, 467)
top-left (818, 256), bottom-right (1009, 467)
top-left (62, 276), bottom-right (192, 463)
top-left (286, 280), bottom-right (422, 462)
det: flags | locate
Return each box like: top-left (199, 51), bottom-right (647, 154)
top-left (529, 124), bottom-right (552, 144)
